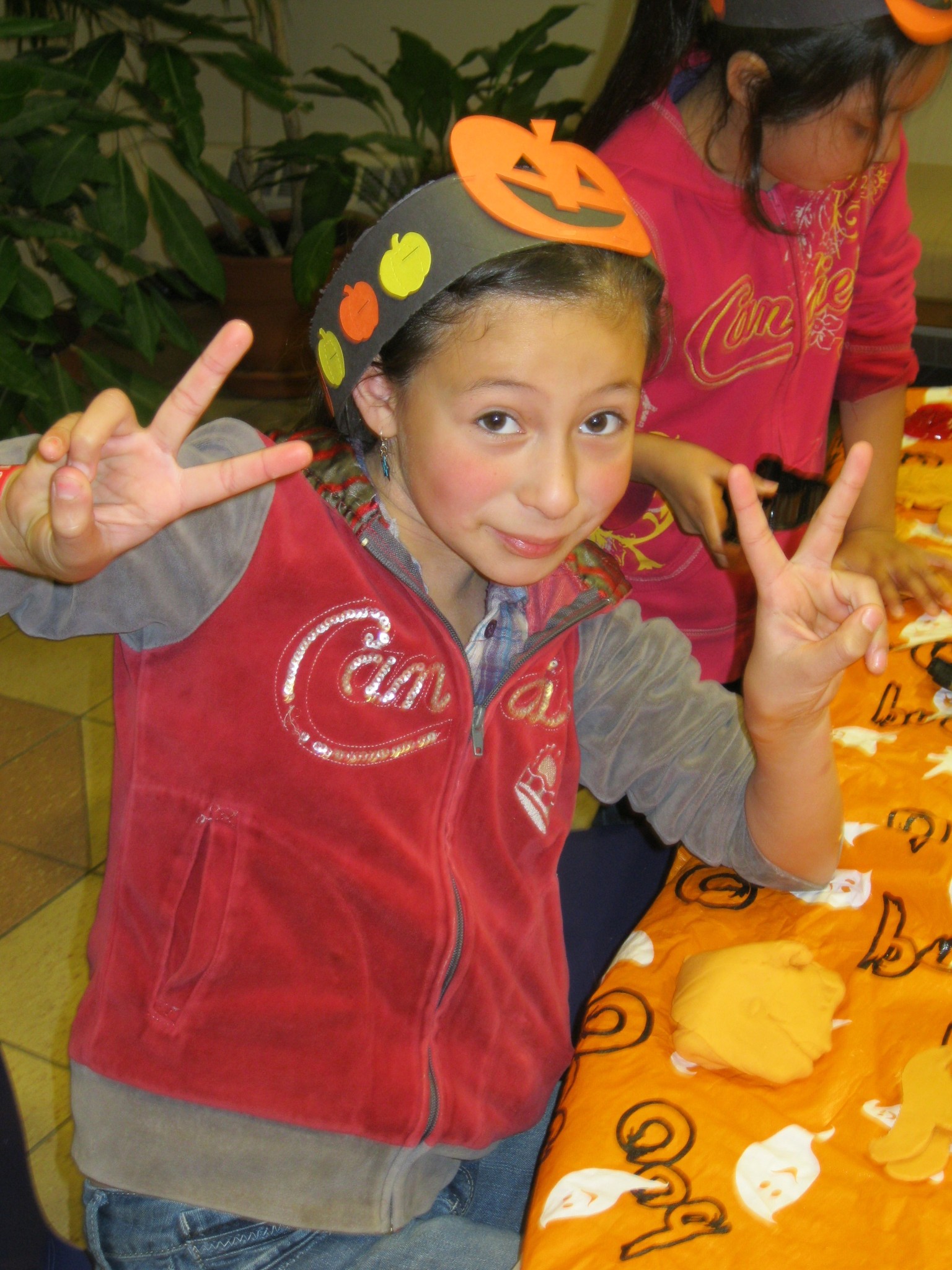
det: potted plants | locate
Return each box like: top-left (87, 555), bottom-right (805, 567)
top-left (2, 2), bottom-right (306, 445)
top-left (186, 1), bottom-right (380, 402)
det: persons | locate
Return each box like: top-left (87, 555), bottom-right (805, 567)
top-left (549, 0), bottom-right (952, 1051)
top-left (0, 116), bottom-right (895, 1268)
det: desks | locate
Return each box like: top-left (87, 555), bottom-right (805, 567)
top-left (506, 385), bottom-right (951, 1269)
